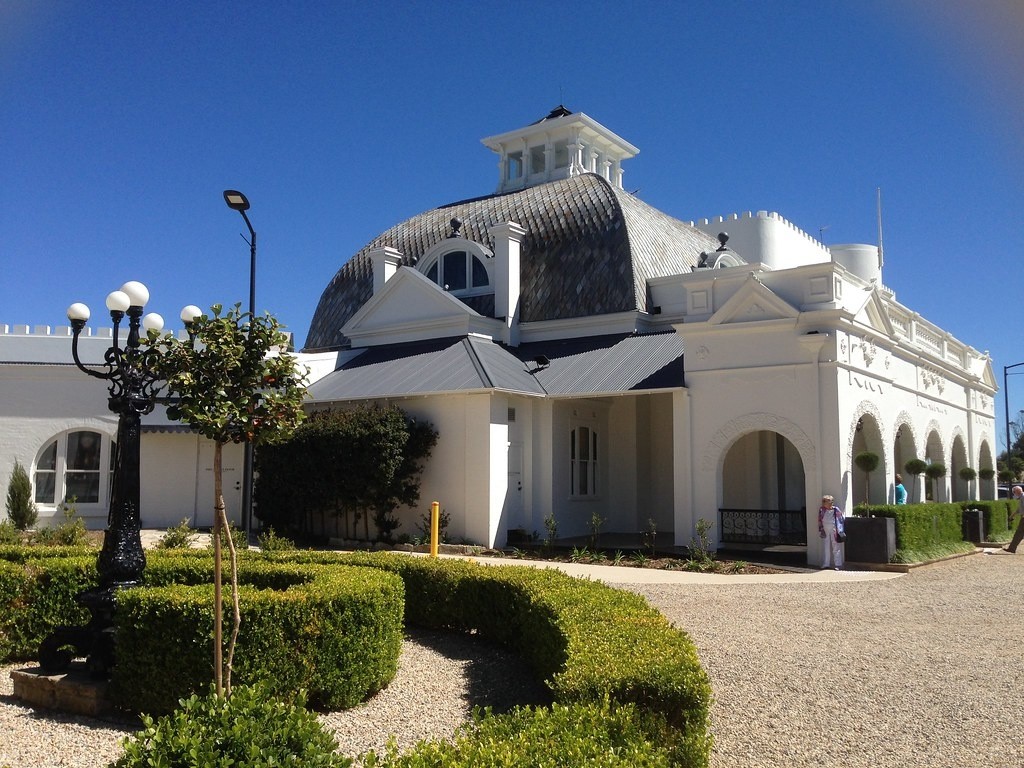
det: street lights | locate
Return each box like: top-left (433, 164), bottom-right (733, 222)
top-left (222, 190), bottom-right (258, 547)
top-left (36, 281), bottom-right (202, 678)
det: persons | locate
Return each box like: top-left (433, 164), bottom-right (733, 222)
top-left (895, 474), bottom-right (908, 505)
top-left (818, 494), bottom-right (847, 571)
top-left (1003, 485), bottom-right (1024, 553)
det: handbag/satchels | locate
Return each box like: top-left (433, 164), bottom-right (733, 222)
top-left (838, 533), bottom-right (846, 542)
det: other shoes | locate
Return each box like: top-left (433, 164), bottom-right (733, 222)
top-left (819, 564), bottom-right (828, 569)
top-left (1004, 548), bottom-right (1015, 553)
top-left (834, 567), bottom-right (839, 571)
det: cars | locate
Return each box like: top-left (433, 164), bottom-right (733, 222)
top-left (998, 487), bottom-right (1018, 501)
